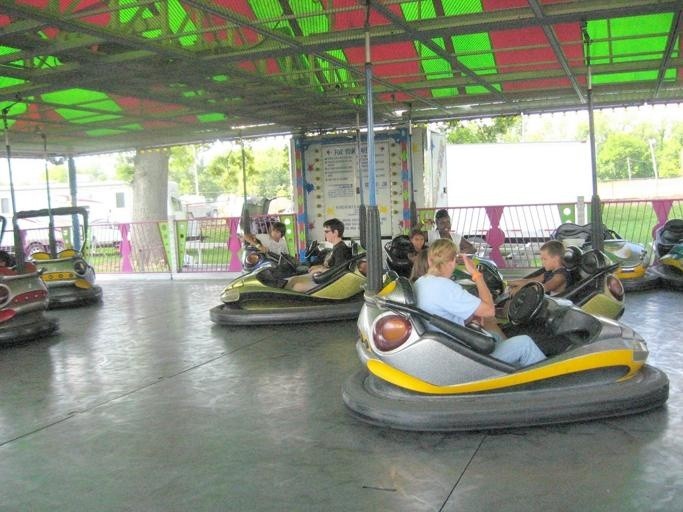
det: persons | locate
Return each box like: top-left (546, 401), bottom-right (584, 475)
top-left (411, 238), bottom-right (549, 368)
top-left (502, 240), bottom-right (569, 318)
top-left (409, 230), bottom-right (425, 255)
top-left (423, 208), bottom-right (477, 263)
top-left (241, 222), bottom-right (288, 262)
top-left (408, 248), bottom-right (507, 342)
top-left (284, 219), bottom-right (348, 292)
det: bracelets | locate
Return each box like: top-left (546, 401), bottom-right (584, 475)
top-left (470, 272), bottom-right (483, 282)
top-left (255, 242), bottom-right (259, 249)
